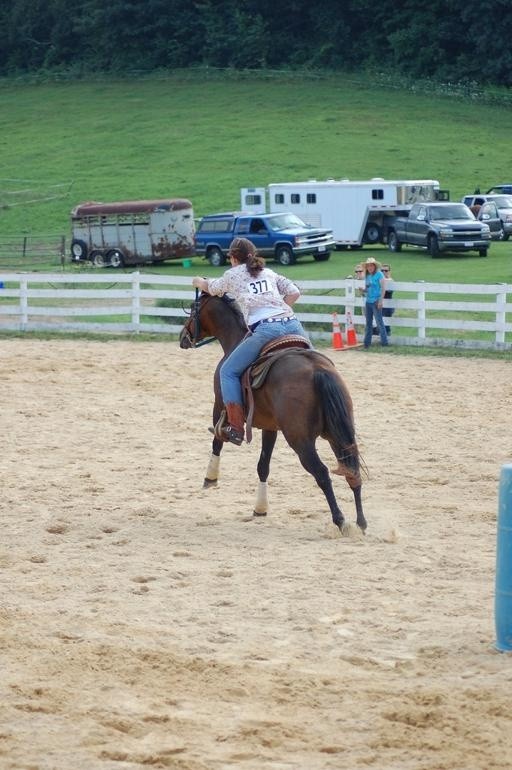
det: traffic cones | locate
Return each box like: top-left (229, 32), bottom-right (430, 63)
top-left (344, 311), bottom-right (365, 348)
top-left (328, 311), bottom-right (349, 352)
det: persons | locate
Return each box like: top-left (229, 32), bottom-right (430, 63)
top-left (346, 264), bottom-right (379, 335)
top-left (190, 235), bottom-right (316, 448)
top-left (379, 264), bottom-right (395, 335)
top-left (469, 202), bottom-right (482, 218)
top-left (358, 257), bottom-right (390, 350)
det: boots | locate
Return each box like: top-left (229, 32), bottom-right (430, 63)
top-left (208, 402), bottom-right (245, 445)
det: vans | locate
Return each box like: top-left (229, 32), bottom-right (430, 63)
top-left (463, 185), bottom-right (512, 240)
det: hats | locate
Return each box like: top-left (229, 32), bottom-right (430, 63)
top-left (361, 258), bottom-right (380, 268)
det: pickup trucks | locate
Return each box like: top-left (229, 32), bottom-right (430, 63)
top-left (382, 201), bottom-right (504, 257)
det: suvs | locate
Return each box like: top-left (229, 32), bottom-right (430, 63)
top-left (193, 212), bottom-right (336, 266)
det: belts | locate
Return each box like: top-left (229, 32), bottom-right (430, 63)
top-left (262, 314), bottom-right (296, 323)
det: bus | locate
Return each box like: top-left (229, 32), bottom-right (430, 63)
top-left (71, 200), bottom-right (198, 268)
top-left (240, 177), bottom-right (449, 254)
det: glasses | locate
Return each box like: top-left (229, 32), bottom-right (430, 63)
top-left (355, 271), bottom-right (362, 273)
top-left (381, 270), bottom-right (388, 272)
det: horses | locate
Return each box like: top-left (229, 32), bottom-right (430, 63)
top-left (178, 289), bottom-right (370, 541)
